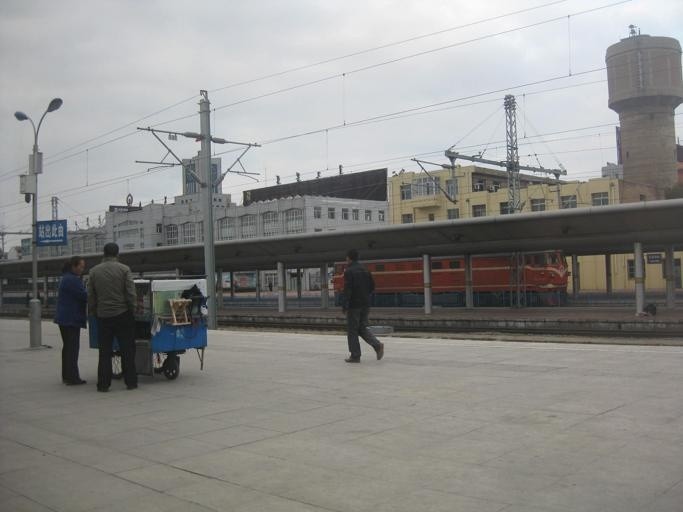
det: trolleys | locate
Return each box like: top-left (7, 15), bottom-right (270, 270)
top-left (89, 279), bottom-right (207, 379)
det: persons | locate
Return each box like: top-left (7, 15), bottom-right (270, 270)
top-left (88, 241), bottom-right (138, 392)
top-left (53, 255), bottom-right (88, 387)
top-left (340, 248), bottom-right (383, 363)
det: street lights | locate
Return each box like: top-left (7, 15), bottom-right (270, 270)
top-left (15, 98), bottom-right (62, 347)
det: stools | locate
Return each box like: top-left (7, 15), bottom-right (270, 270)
top-left (167, 297), bottom-right (192, 326)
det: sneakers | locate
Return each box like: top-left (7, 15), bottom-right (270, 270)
top-left (343, 355), bottom-right (360, 363)
top-left (376, 343), bottom-right (384, 361)
top-left (63, 379), bottom-right (138, 392)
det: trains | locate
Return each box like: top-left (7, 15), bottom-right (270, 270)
top-left (332, 250), bottom-right (569, 307)
